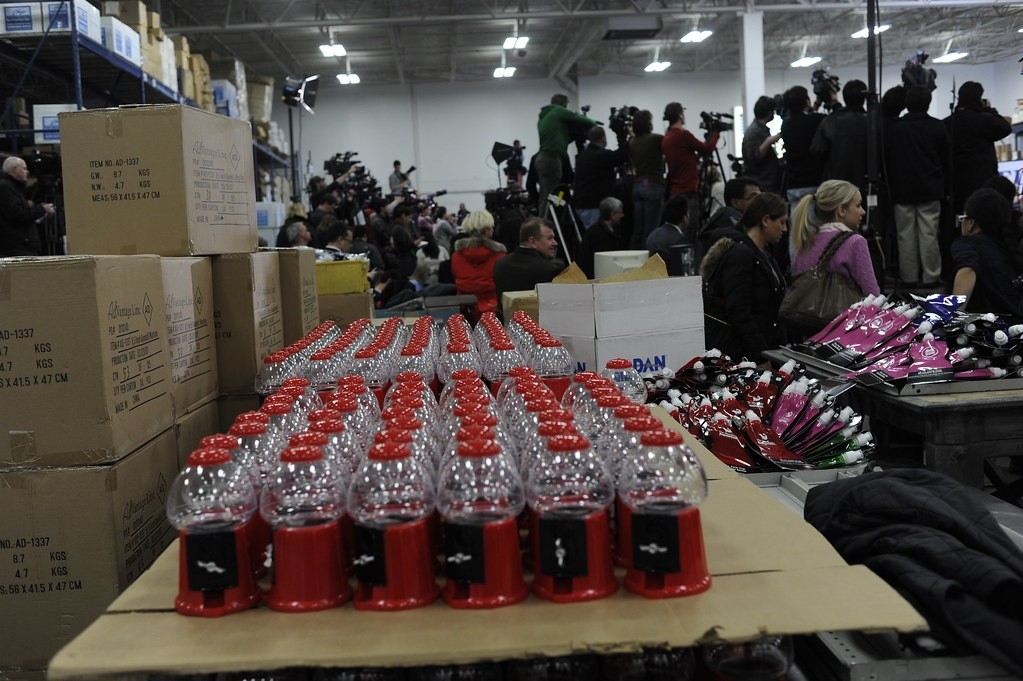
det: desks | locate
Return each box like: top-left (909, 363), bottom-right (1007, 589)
top-left (47, 405), bottom-right (929, 681)
top-left (759, 346), bottom-right (1023, 515)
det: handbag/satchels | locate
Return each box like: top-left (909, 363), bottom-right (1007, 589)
top-left (776, 230), bottom-right (863, 328)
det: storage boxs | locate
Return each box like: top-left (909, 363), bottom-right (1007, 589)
top-left (503, 275), bottom-right (708, 383)
top-left (0, 0), bottom-right (372, 681)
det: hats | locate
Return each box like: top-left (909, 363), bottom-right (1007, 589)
top-left (309, 176), bottom-right (325, 185)
top-left (664, 103), bottom-right (686, 117)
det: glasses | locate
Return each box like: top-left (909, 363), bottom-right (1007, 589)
top-left (955, 214), bottom-right (968, 222)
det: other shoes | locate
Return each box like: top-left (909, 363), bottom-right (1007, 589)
top-left (893, 279), bottom-right (949, 290)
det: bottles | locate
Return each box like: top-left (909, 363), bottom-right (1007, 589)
top-left (160, 635), bottom-right (795, 681)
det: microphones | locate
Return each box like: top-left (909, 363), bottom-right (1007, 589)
top-left (724, 113), bottom-right (734, 118)
top-left (352, 153), bottom-right (358, 155)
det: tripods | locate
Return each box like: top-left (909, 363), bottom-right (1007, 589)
top-left (698, 133), bottom-right (726, 220)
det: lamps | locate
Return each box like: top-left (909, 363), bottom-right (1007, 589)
top-left (336, 57), bottom-right (361, 85)
top-left (492, 18), bottom-right (530, 78)
top-left (317, 15), bottom-right (348, 58)
top-left (642, 14), bottom-right (892, 74)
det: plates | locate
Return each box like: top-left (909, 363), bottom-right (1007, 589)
top-left (166, 310), bottom-right (711, 619)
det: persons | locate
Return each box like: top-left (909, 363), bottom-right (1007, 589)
top-left (700, 192), bottom-right (789, 373)
top-left (0, 79), bottom-right (1023, 326)
top-left (788, 179), bottom-right (880, 346)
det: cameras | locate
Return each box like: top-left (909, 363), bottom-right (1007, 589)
top-left (401, 166), bottom-right (415, 180)
